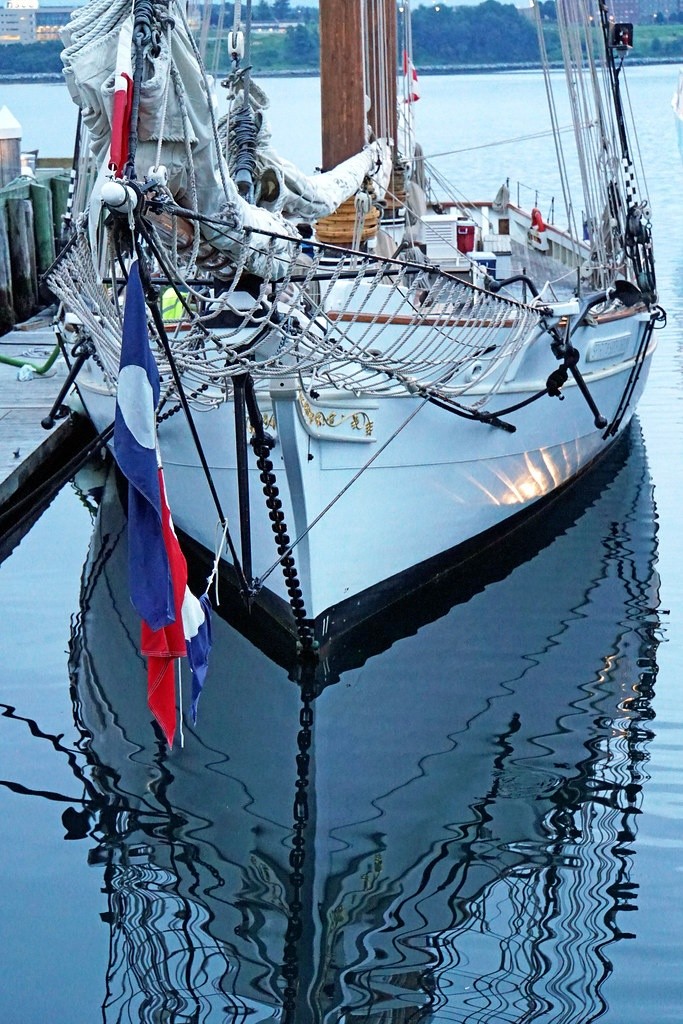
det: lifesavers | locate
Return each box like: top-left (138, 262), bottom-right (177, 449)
top-left (531, 208), bottom-right (545, 232)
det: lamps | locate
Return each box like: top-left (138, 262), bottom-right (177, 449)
top-left (0, 106), bottom-right (23, 141)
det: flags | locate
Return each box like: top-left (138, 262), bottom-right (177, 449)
top-left (113, 247), bottom-right (213, 752)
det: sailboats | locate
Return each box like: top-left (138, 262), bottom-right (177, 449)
top-left (4, 418), bottom-right (677, 1024)
top-left (41, 0), bottom-right (670, 653)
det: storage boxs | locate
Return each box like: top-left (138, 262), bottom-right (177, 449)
top-left (457, 220), bottom-right (475, 253)
top-left (468, 251), bottom-right (498, 285)
